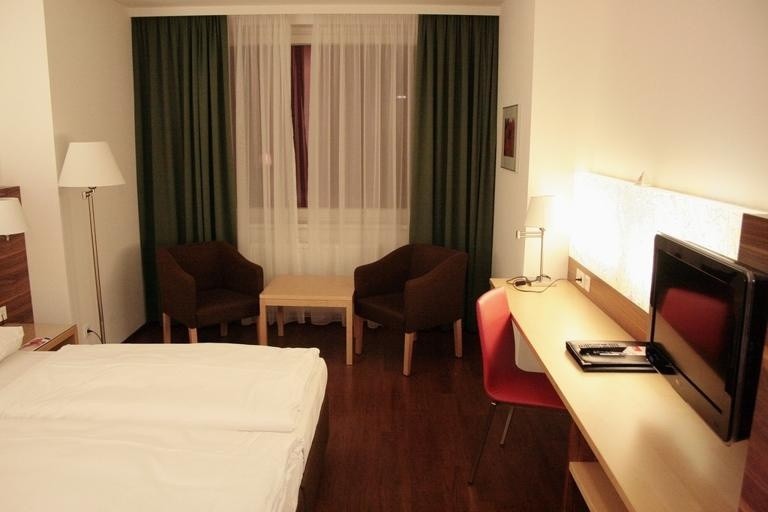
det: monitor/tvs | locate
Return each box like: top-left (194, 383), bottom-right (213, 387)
top-left (646, 231), bottom-right (768, 451)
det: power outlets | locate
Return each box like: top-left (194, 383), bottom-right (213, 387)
top-left (572, 267), bottom-right (591, 294)
top-left (84, 325), bottom-right (91, 337)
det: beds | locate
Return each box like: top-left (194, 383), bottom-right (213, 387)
top-left (0, 340), bottom-right (331, 512)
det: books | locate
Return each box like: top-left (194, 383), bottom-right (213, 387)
top-left (565, 337), bottom-right (657, 374)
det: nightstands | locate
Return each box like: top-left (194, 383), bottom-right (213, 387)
top-left (0, 319), bottom-right (82, 352)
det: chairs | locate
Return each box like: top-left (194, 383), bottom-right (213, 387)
top-left (467, 286), bottom-right (572, 490)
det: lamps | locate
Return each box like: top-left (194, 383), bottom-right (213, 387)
top-left (522, 193), bottom-right (558, 283)
top-left (0, 195), bottom-right (31, 241)
top-left (55, 136), bottom-right (129, 348)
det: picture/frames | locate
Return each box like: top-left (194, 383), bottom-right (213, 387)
top-left (499, 101), bottom-right (518, 176)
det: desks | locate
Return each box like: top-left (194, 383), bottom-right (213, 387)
top-left (255, 274), bottom-right (366, 368)
top-left (489, 256), bottom-right (748, 512)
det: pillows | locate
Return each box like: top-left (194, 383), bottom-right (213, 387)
top-left (0, 324), bottom-right (24, 364)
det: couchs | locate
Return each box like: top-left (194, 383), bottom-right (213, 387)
top-left (153, 237), bottom-right (263, 343)
top-left (350, 239), bottom-right (465, 375)
